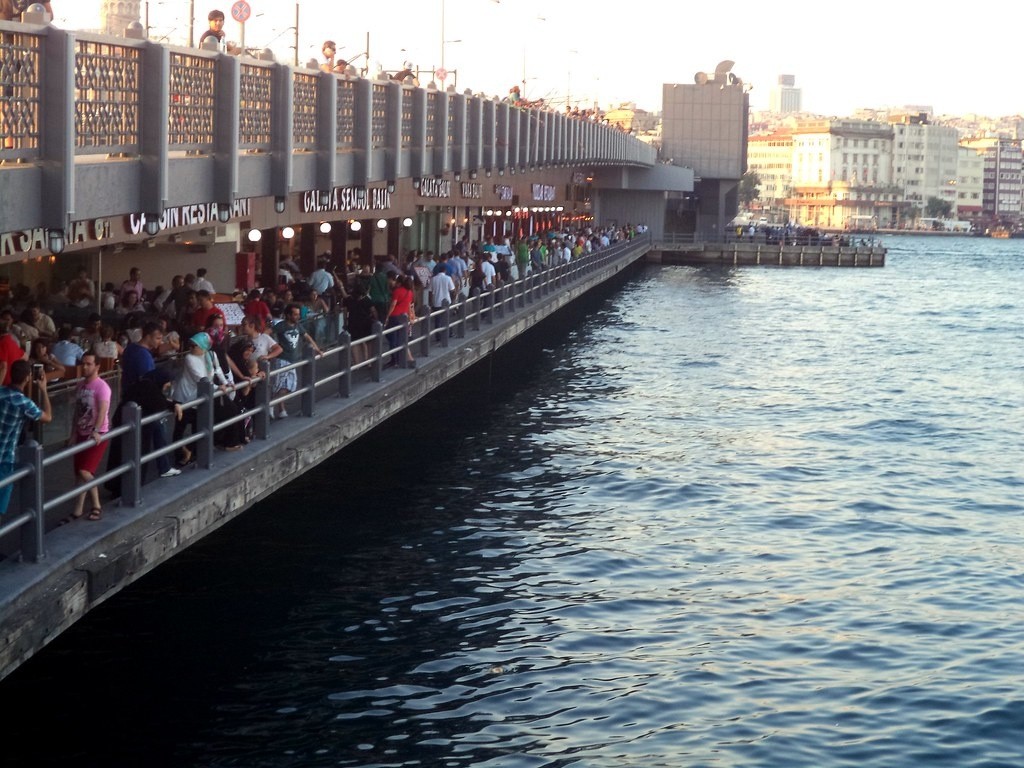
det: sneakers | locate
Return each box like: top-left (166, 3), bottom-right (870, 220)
top-left (161, 467), bottom-right (181, 477)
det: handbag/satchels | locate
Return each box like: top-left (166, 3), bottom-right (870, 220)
top-left (209, 350), bottom-right (220, 404)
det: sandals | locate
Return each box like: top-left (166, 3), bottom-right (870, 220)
top-left (60, 512), bottom-right (83, 526)
top-left (88, 507), bottom-right (103, 520)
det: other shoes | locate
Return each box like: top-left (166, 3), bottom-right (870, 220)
top-left (181, 450), bottom-right (191, 465)
top-left (278, 410), bottom-right (287, 418)
top-left (269, 406), bottom-right (275, 419)
top-left (226, 445), bottom-right (243, 451)
top-left (407, 360), bottom-right (416, 368)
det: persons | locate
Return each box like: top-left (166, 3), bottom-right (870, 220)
top-left (195, 9), bottom-right (241, 68)
top-left (749, 226), bottom-right (755, 243)
top-left (381, 275), bottom-right (414, 369)
top-left (242, 290), bottom-right (272, 334)
top-left (170, 332), bottom-right (237, 467)
top-left (426, 266), bottom-right (455, 342)
top-left (118, 265), bottom-right (144, 307)
top-left (318, 39), bottom-right (338, 73)
top-left (0, 223), bottom-right (648, 505)
top-left (392, 60), bottom-right (420, 87)
top-left (308, 261), bottom-right (336, 297)
top-left (0, 359), bottom-right (53, 521)
top-left (455, 235), bottom-right (468, 258)
top-left (504, 86), bottom-right (633, 135)
top-left (735, 225), bottom-right (743, 242)
top-left (748, 221), bottom-right (872, 247)
top-left (333, 60), bottom-right (355, 142)
top-left (58, 352), bottom-right (111, 528)
top-left (192, 268), bottom-right (216, 293)
top-left (0, 0), bottom-right (54, 150)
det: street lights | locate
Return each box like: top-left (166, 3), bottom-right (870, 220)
top-left (522, 17), bottom-right (547, 100)
top-left (441, 39), bottom-right (461, 91)
